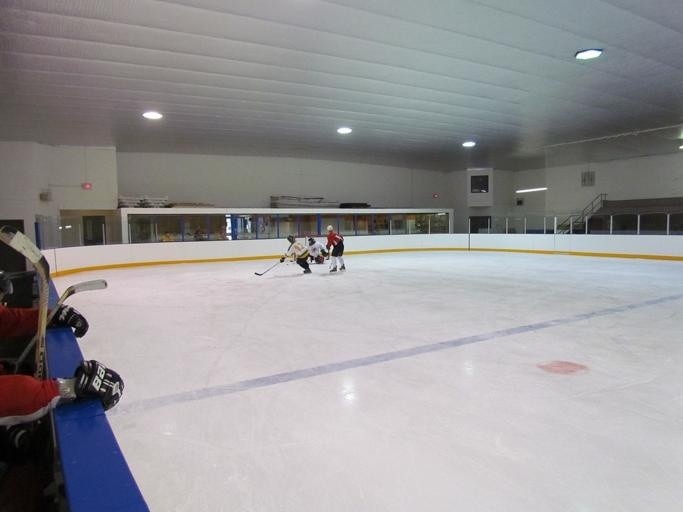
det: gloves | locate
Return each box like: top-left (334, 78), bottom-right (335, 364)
top-left (46, 304), bottom-right (88, 337)
top-left (280, 258), bottom-right (285, 263)
top-left (75, 360), bottom-right (124, 411)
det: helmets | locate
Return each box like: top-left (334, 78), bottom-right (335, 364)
top-left (308, 238), bottom-right (316, 246)
top-left (287, 235), bottom-right (295, 242)
top-left (327, 225), bottom-right (333, 231)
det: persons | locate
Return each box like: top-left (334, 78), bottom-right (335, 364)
top-left (326, 225), bottom-right (345, 272)
top-left (0, 302), bottom-right (125, 426)
top-left (280, 234), bottom-right (312, 274)
top-left (308, 238), bottom-right (327, 263)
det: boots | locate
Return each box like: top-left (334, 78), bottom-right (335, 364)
top-left (339, 264), bottom-right (345, 270)
top-left (330, 266), bottom-right (337, 272)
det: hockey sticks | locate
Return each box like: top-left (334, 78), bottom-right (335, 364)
top-left (0, 226), bottom-right (107, 483)
top-left (254, 261), bottom-right (280, 276)
top-left (326, 250), bottom-right (330, 260)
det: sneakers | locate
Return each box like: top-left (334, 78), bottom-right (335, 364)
top-left (304, 269), bottom-right (311, 273)
